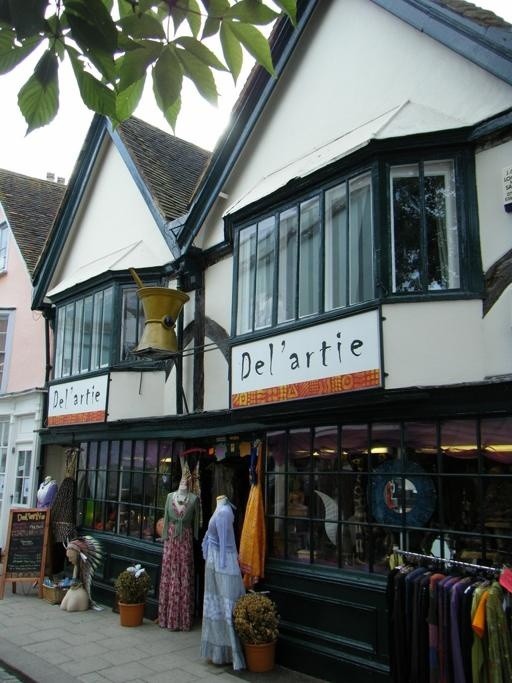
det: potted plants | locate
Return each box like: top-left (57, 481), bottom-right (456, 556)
top-left (233, 592), bottom-right (281, 673)
top-left (114, 568), bottom-right (152, 627)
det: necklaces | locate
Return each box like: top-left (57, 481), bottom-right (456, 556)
top-left (42, 478), bottom-right (53, 488)
top-left (176, 491), bottom-right (189, 505)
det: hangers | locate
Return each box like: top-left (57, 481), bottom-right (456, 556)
top-left (398, 550), bottom-right (499, 595)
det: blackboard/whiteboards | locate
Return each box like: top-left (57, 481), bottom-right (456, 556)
top-left (5, 512), bottom-right (47, 578)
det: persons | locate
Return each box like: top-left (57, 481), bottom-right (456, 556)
top-left (201, 492), bottom-right (248, 666)
top-left (154, 483), bottom-right (200, 632)
top-left (286, 476), bottom-right (305, 507)
top-left (34, 472), bottom-right (57, 510)
top-left (57, 538), bottom-right (98, 614)
